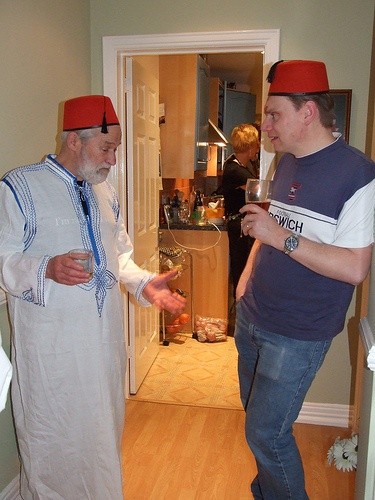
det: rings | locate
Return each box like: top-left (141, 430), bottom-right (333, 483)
top-left (246, 223), bottom-right (251, 229)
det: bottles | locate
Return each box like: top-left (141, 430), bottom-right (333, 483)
top-left (190, 186), bottom-right (196, 202)
top-left (193, 189), bottom-right (204, 216)
top-left (163, 187), bottom-right (189, 223)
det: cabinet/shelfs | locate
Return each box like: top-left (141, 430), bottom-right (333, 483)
top-left (159, 51), bottom-right (258, 179)
top-left (159, 227), bottom-right (231, 338)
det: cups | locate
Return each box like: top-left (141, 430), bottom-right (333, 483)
top-left (246, 179), bottom-right (273, 216)
top-left (68, 249), bottom-right (94, 281)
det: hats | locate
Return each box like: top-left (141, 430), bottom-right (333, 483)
top-left (62, 94), bottom-right (121, 135)
top-left (265, 59), bottom-right (329, 96)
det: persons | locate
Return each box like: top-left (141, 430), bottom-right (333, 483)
top-left (222, 123), bottom-right (260, 298)
top-left (234, 58), bottom-right (375, 500)
top-left (0, 96), bottom-right (188, 500)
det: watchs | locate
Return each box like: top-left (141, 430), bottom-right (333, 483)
top-left (282, 232), bottom-right (301, 255)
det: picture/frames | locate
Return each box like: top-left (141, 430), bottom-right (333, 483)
top-left (130, 332), bottom-right (244, 411)
top-left (329, 88), bottom-right (353, 144)
